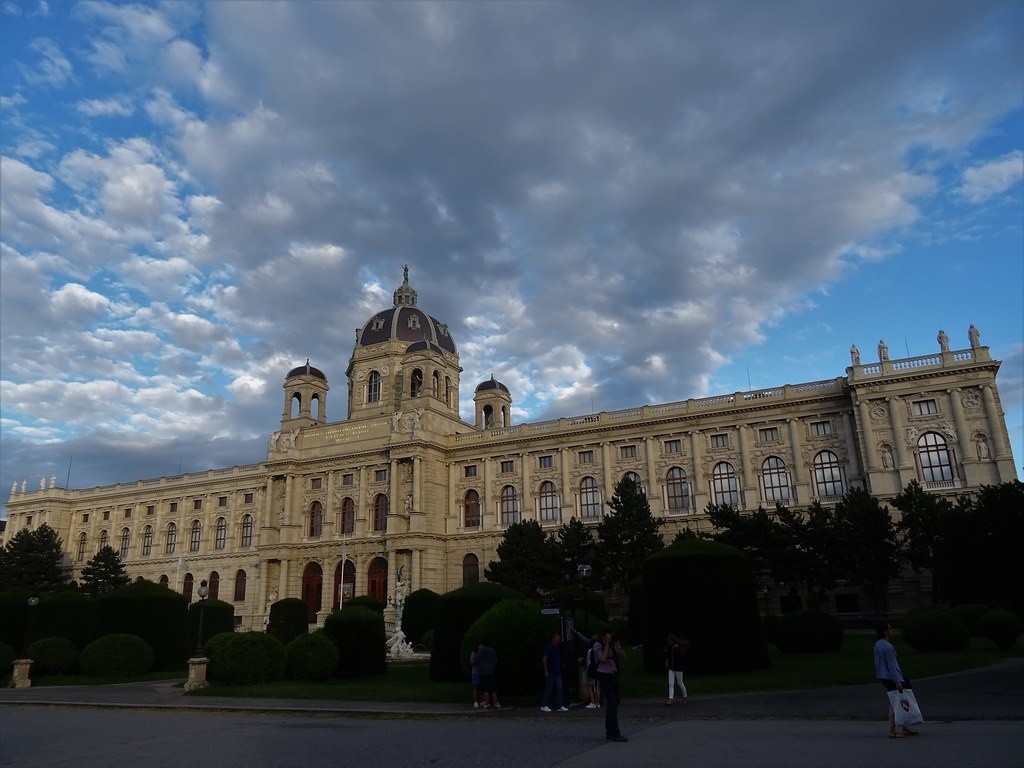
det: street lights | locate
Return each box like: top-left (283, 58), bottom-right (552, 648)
top-left (578, 564), bottom-right (593, 631)
top-left (28, 597), bottom-right (39, 640)
top-left (195, 580), bottom-right (208, 658)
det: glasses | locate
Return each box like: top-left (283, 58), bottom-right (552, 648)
top-left (889, 626), bottom-right (893, 630)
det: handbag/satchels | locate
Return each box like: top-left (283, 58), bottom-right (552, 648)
top-left (887, 689), bottom-right (923, 727)
top-left (582, 669), bottom-right (595, 688)
top-left (665, 657), bottom-right (669, 669)
top-left (901, 676), bottom-right (912, 689)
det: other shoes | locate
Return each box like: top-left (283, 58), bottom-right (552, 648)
top-left (683, 697), bottom-right (688, 704)
top-left (479, 704), bottom-right (490, 712)
top-left (606, 734), bottom-right (628, 742)
top-left (494, 703), bottom-right (502, 710)
top-left (667, 700), bottom-right (673, 705)
top-left (889, 731), bottom-right (906, 738)
top-left (902, 729), bottom-right (921, 736)
top-left (474, 701), bottom-right (485, 707)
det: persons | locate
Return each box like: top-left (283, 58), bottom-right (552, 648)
top-left (540, 634), bottom-right (568, 712)
top-left (657, 633), bottom-right (693, 705)
top-left (390, 411), bottom-right (420, 431)
top-left (385, 580), bottom-right (414, 657)
top-left (976, 438), bottom-right (989, 459)
top-left (968, 324), bottom-right (979, 348)
top-left (401, 264), bottom-right (409, 282)
top-left (270, 429), bottom-right (296, 449)
top-left (470, 643), bottom-right (504, 712)
top-left (877, 339), bottom-right (889, 360)
top-left (881, 448), bottom-right (894, 470)
top-left (569, 628), bottom-right (628, 742)
top-left (937, 329), bottom-right (950, 352)
top-left (873, 622), bottom-right (924, 738)
top-left (851, 344), bottom-right (860, 365)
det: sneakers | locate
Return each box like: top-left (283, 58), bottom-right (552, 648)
top-left (541, 706), bottom-right (551, 711)
top-left (585, 703), bottom-right (600, 708)
top-left (557, 706), bottom-right (568, 711)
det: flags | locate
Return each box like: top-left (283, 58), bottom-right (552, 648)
top-left (180, 558), bottom-right (189, 570)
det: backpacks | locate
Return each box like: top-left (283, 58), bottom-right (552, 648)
top-left (588, 641), bottom-right (619, 678)
